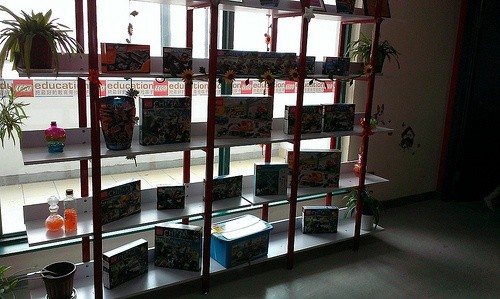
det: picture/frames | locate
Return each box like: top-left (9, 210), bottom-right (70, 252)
top-left (363, 0), bottom-right (391, 18)
top-left (301, 0), bottom-right (327, 12)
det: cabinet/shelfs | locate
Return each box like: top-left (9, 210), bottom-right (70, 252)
top-left (13, 0), bottom-right (396, 299)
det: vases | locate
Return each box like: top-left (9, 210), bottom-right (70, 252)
top-left (97, 93), bottom-right (134, 151)
top-left (39, 260), bottom-right (77, 299)
top-left (336, 0), bottom-right (356, 14)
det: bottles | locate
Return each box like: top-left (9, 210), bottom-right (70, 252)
top-left (45, 196), bottom-right (64, 231)
top-left (353, 153), bottom-right (368, 176)
top-left (44, 122), bottom-right (65, 152)
top-left (63, 189), bottom-right (78, 232)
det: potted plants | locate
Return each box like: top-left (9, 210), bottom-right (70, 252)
top-left (345, 29), bottom-right (402, 74)
top-left (343, 187), bottom-right (384, 232)
top-left (0, 7), bottom-right (84, 148)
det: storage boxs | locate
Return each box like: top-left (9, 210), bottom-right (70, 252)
top-left (155, 185), bottom-right (186, 210)
top-left (254, 162), bottom-right (288, 196)
top-left (296, 55), bottom-right (316, 75)
top-left (324, 55), bottom-right (351, 75)
top-left (217, 49), bottom-right (257, 76)
top-left (257, 52), bottom-right (296, 75)
top-left (287, 147), bottom-right (341, 188)
top-left (102, 237), bottom-right (150, 291)
top-left (281, 102), bottom-right (324, 135)
top-left (323, 103), bottom-right (356, 133)
top-left (101, 179), bottom-right (142, 226)
top-left (203, 175), bottom-right (246, 202)
top-left (214, 93), bottom-right (274, 139)
top-left (301, 206), bottom-right (339, 234)
top-left (162, 46), bottom-right (193, 74)
top-left (153, 223), bottom-right (202, 272)
top-left (210, 212), bottom-right (275, 270)
top-left (138, 95), bottom-right (193, 145)
top-left (100, 43), bottom-right (150, 74)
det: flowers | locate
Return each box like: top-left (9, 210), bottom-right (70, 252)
top-left (262, 71), bottom-right (274, 86)
top-left (363, 64), bottom-right (375, 76)
top-left (288, 68), bottom-right (299, 81)
top-left (181, 69), bottom-right (194, 84)
top-left (224, 70), bottom-right (236, 84)
